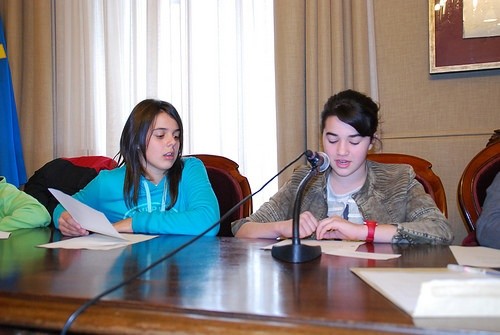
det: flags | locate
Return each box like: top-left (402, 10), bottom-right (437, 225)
top-left (0, 17), bottom-right (28, 188)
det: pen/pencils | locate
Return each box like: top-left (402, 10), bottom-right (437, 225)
top-left (275, 235), bottom-right (286, 240)
top-left (447, 264), bottom-right (500, 276)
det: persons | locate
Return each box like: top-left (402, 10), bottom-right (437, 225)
top-left (0, 176), bottom-right (51, 231)
top-left (53, 99), bottom-right (221, 236)
top-left (477, 173), bottom-right (500, 248)
top-left (231, 90), bottom-right (454, 244)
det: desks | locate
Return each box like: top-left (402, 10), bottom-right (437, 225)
top-left (0, 227), bottom-right (500, 335)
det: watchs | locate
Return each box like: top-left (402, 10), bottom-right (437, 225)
top-left (363, 220), bottom-right (378, 243)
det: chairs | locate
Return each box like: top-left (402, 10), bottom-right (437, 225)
top-left (458, 129), bottom-right (500, 246)
top-left (183, 154), bottom-right (254, 237)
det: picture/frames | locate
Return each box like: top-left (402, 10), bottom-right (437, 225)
top-left (429, 0), bottom-right (500, 74)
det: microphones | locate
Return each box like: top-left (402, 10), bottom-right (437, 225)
top-left (305, 150), bottom-right (330, 172)
